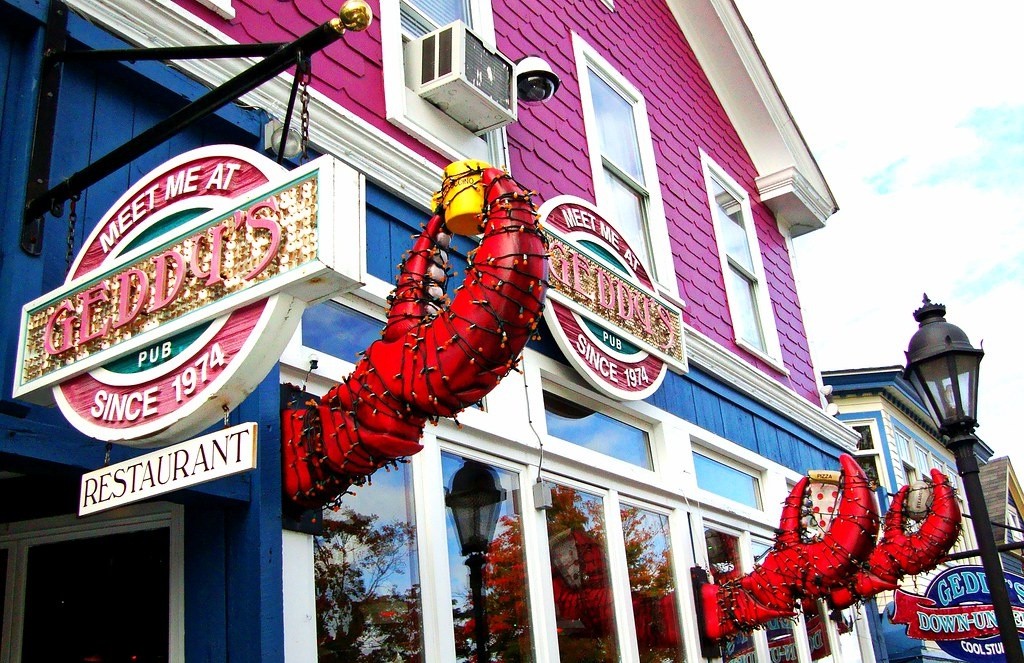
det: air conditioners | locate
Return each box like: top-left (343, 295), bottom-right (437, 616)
top-left (408, 18), bottom-right (520, 138)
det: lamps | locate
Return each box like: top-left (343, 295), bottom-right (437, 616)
top-left (262, 119), bottom-right (303, 159)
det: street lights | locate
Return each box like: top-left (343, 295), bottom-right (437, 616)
top-left (443, 458), bottom-right (507, 663)
top-left (902, 290), bottom-right (1024, 663)
